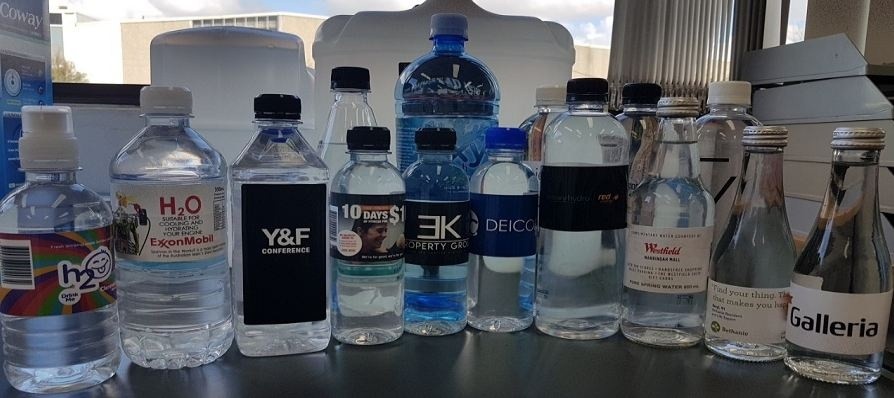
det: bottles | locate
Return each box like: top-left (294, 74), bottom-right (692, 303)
top-left (0, 11), bottom-right (893, 384)
